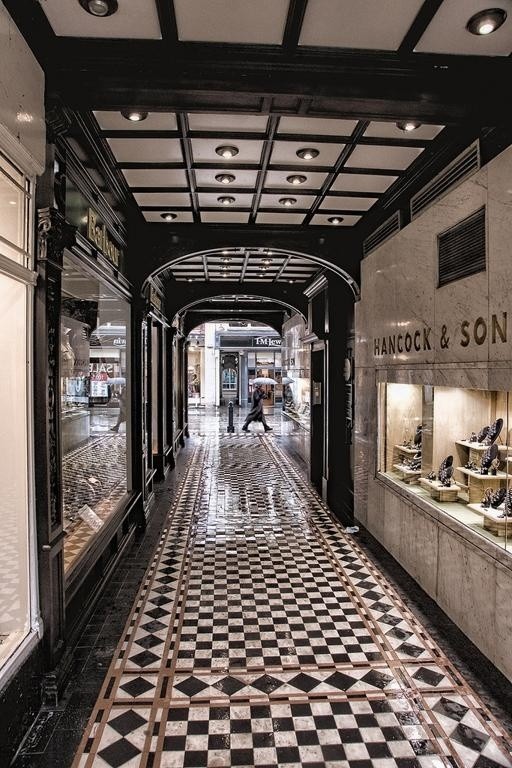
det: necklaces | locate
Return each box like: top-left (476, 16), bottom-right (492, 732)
top-left (399, 419), bottom-right (512, 516)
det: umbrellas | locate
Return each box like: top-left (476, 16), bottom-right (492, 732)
top-left (106, 376), bottom-right (126, 386)
top-left (250, 377), bottom-right (279, 385)
top-left (282, 377), bottom-right (295, 386)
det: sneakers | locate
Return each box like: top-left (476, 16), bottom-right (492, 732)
top-left (265, 428), bottom-right (273, 432)
top-left (242, 427), bottom-right (250, 432)
top-left (110, 426), bottom-right (119, 433)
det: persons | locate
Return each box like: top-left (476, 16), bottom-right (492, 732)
top-left (241, 383), bottom-right (273, 432)
top-left (109, 385), bottom-right (126, 430)
top-left (283, 384), bottom-right (301, 430)
